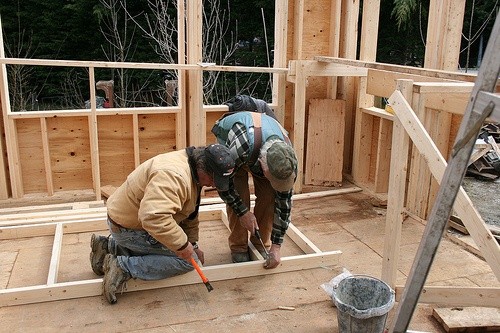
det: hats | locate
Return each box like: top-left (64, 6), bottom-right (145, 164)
top-left (204, 144), bottom-right (236, 192)
top-left (266, 142), bottom-right (297, 192)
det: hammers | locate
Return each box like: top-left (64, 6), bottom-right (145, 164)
top-left (191, 259), bottom-right (213, 292)
top-left (255, 230), bottom-right (275, 266)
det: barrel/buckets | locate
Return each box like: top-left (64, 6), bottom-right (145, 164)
top-left (331, 274), bottom-right (395, 333)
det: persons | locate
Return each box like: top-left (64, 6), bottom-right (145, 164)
top-left (211, 95), bottom-right (299, 269)
top-left (90, 144), bottom-right (234, 305)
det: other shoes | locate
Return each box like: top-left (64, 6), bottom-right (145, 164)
top-left (103, 253), bottom-right (126, 305)
top-left (89, 233), bottom-right (110, 277)
top-left (252, 242), bottom-right (271, 259)
top-left (231, 250), bottom-right (250, 263)
